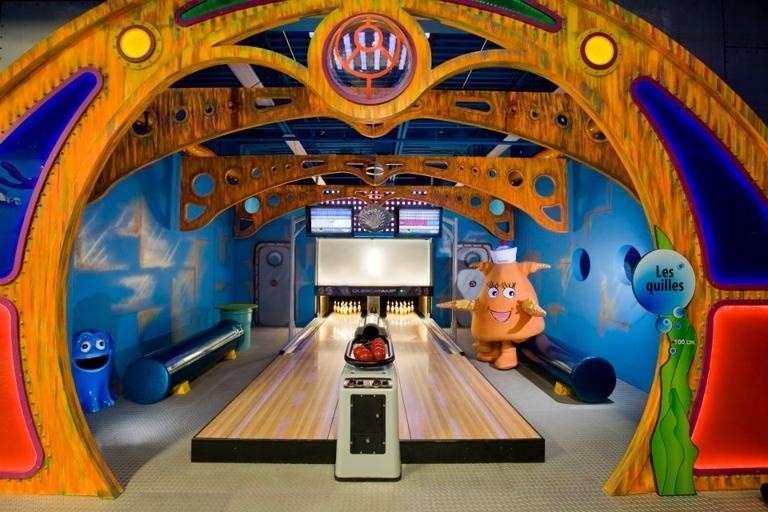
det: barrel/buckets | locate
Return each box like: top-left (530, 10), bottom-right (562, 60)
top-left (218, 310), bottom-right (253, 352)
